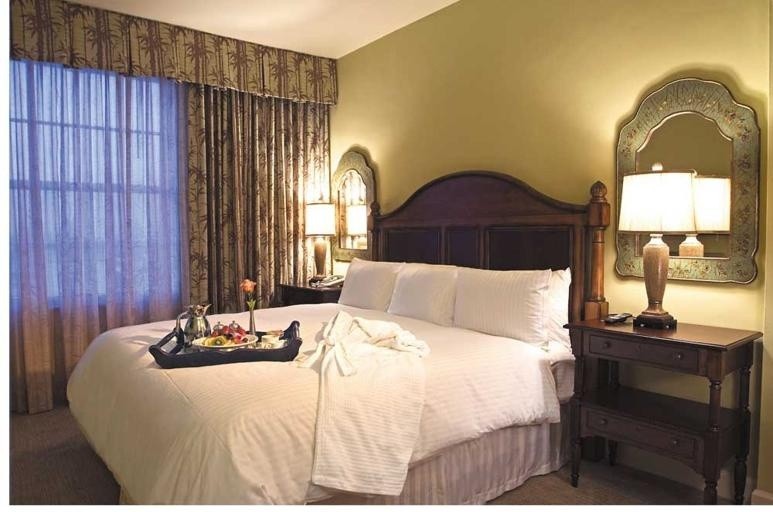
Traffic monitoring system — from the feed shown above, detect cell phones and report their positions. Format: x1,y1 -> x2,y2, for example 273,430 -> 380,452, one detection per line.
604,312 -> 632,324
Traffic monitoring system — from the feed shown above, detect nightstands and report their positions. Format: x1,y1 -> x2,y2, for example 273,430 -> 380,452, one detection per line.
275,282 -> 341,305
561,313 -> 763,504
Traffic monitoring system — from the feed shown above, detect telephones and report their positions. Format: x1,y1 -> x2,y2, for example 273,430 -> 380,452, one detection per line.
319,273 -> 345,289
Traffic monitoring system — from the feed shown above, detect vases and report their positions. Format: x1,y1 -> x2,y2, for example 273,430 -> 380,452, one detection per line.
248,310 -> 256,335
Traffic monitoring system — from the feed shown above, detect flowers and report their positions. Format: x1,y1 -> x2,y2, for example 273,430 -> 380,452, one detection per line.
238,278 -> 256,309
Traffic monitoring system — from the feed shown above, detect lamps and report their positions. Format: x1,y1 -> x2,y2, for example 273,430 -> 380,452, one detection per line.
344,205 -> 366,250
304,202 -> 336,283
617,170 -> 697,329
678,174 -> 731,258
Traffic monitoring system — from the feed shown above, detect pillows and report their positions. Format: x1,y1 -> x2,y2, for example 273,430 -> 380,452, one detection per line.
550,266 -> 572,342
453,267 -> 552,344
337,258 -> 402,311
387,263 -> 455,326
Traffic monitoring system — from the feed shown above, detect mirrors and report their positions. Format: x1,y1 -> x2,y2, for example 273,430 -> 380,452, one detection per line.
330,150 -> 375,262
613,78 -> 761,284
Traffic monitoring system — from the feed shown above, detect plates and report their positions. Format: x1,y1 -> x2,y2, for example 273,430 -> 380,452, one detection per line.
191,334 -> 258,348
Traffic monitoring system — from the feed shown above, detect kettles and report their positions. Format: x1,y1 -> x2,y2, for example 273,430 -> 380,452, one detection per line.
176,303 -> 212,346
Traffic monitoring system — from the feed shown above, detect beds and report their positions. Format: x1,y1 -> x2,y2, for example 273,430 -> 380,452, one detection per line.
66,171 -> 612,505
